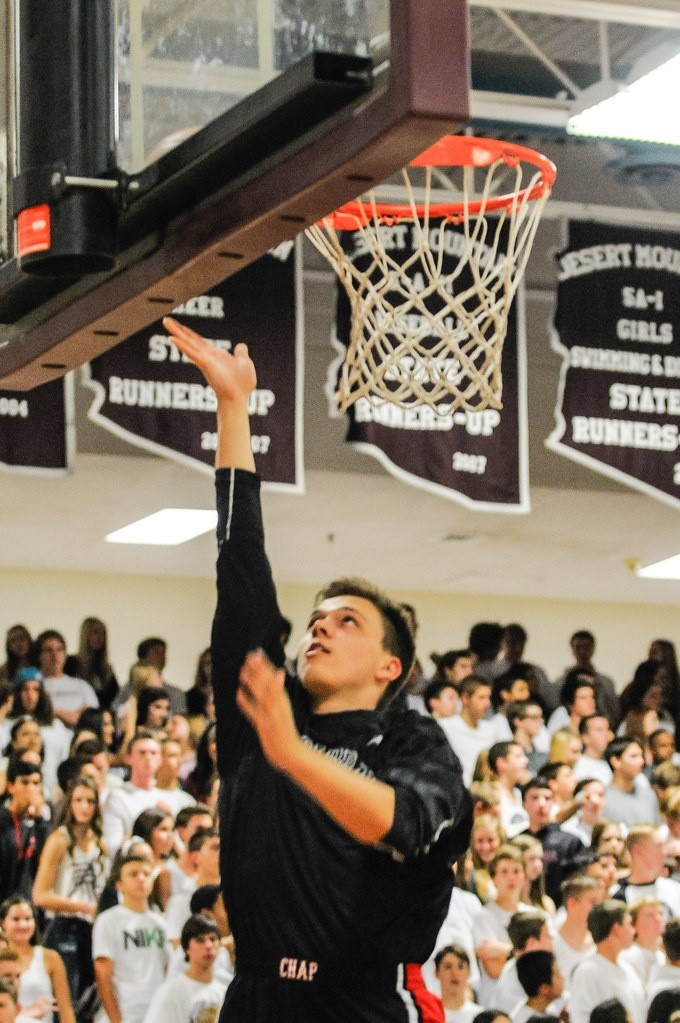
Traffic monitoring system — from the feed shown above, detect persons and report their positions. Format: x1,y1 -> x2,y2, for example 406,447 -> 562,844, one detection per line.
161,309 -> 469,1023
0,600 -> 680,1023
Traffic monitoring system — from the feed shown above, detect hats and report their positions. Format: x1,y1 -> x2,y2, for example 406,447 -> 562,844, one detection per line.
13,666 -> 42,686
